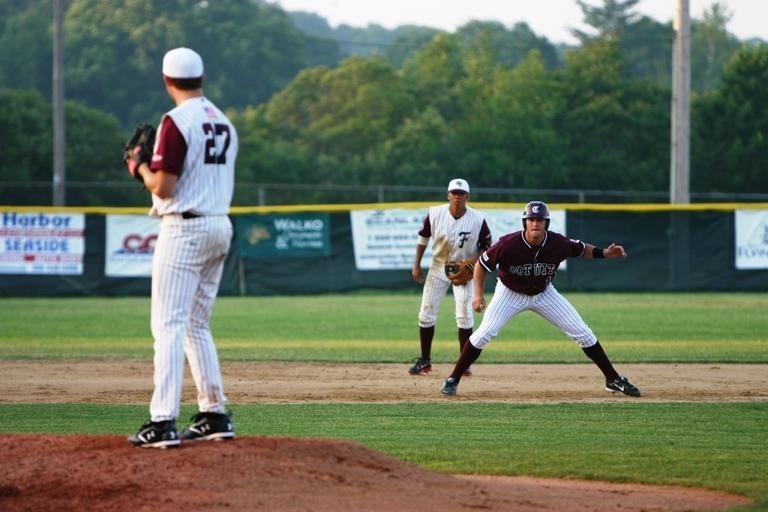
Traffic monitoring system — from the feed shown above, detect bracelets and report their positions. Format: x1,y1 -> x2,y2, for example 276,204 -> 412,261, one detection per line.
592,246 -> 606,258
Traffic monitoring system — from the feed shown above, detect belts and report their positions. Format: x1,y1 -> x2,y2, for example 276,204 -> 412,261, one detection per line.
181,210 -> 203,219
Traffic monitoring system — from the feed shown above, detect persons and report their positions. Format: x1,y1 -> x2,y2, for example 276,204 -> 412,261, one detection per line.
440,201 -> 641,399
124,46 -> 239,448
408,178 -> 492,377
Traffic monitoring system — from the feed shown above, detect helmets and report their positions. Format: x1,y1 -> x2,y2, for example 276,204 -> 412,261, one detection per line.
522,201 -> 550,230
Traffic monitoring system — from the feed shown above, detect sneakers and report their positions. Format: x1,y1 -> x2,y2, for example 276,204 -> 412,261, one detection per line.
179,411 -> 236,441
409,358 -> 432,374
440,377 -> 459,395
128,420 -> 181,448
605,376 -> 641,397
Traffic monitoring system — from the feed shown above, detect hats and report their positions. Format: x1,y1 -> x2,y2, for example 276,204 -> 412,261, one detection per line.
448,178 -> 470,194
162,46 -> 203,79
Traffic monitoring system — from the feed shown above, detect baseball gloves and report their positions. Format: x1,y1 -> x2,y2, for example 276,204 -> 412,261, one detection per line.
123,123 -> 155,184
445,255 -> 478,286
